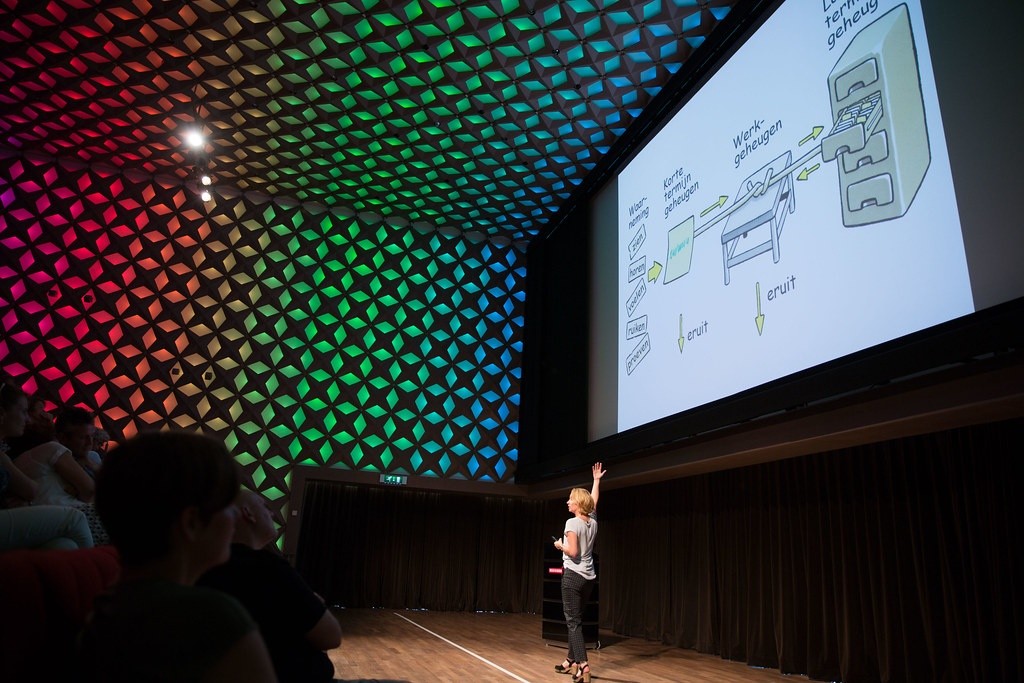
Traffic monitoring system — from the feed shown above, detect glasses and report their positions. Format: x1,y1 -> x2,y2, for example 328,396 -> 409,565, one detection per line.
0,377 -> 20,392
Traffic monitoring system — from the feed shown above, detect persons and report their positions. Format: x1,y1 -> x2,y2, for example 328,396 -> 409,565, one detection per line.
554,462 -> 607,683
0,382 -> 410,683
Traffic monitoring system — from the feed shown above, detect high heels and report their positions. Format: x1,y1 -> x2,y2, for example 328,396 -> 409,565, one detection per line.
555,659 -> 576,674
572,665 -> 591,683
